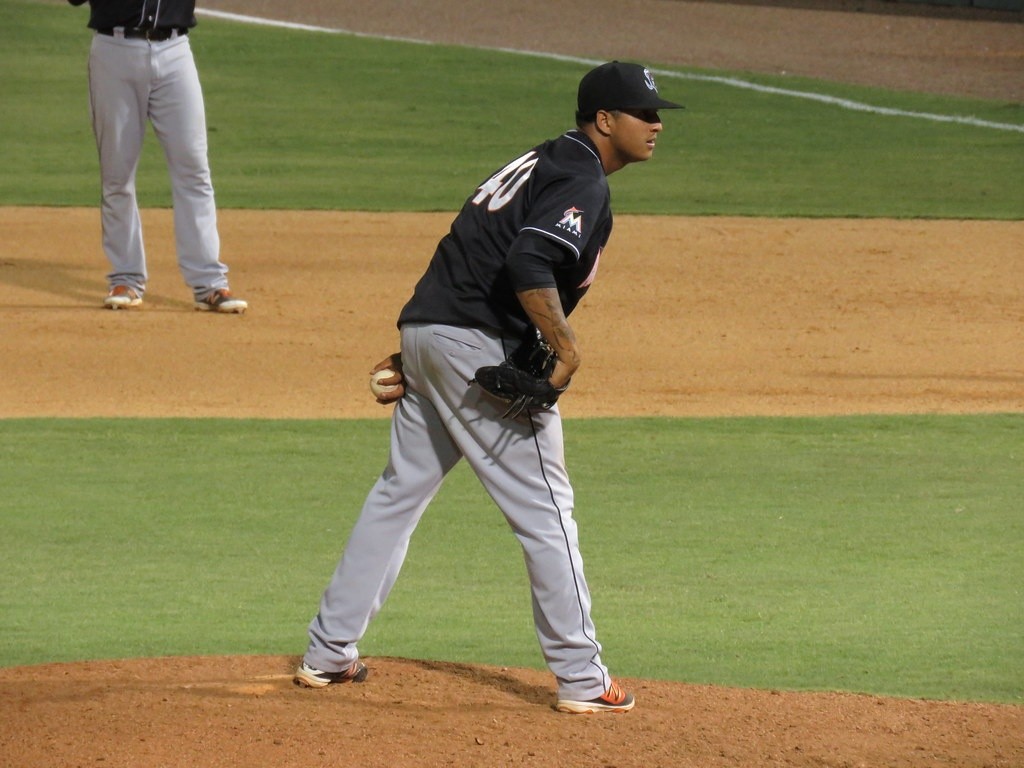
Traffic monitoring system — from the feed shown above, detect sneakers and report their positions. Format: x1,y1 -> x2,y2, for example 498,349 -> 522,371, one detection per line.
293,661 -> 367,688
104,286 -> 142,307
194,289 -> 248,312
557,681 -> 635,714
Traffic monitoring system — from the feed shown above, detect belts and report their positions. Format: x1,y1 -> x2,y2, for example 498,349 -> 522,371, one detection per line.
97,26 -> 188,42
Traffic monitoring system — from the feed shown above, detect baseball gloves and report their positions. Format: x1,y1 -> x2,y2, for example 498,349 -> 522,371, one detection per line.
467,338 -> 571,419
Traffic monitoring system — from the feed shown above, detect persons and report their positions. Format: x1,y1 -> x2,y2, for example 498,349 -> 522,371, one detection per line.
292,59 -> 685,714
67,0 -> 249,313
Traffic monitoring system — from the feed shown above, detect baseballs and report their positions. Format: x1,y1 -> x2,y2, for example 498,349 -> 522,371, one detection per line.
369,368 -> 401,399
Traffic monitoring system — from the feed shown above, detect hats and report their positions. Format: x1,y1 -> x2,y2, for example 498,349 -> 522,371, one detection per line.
578,60 -> 686,112
